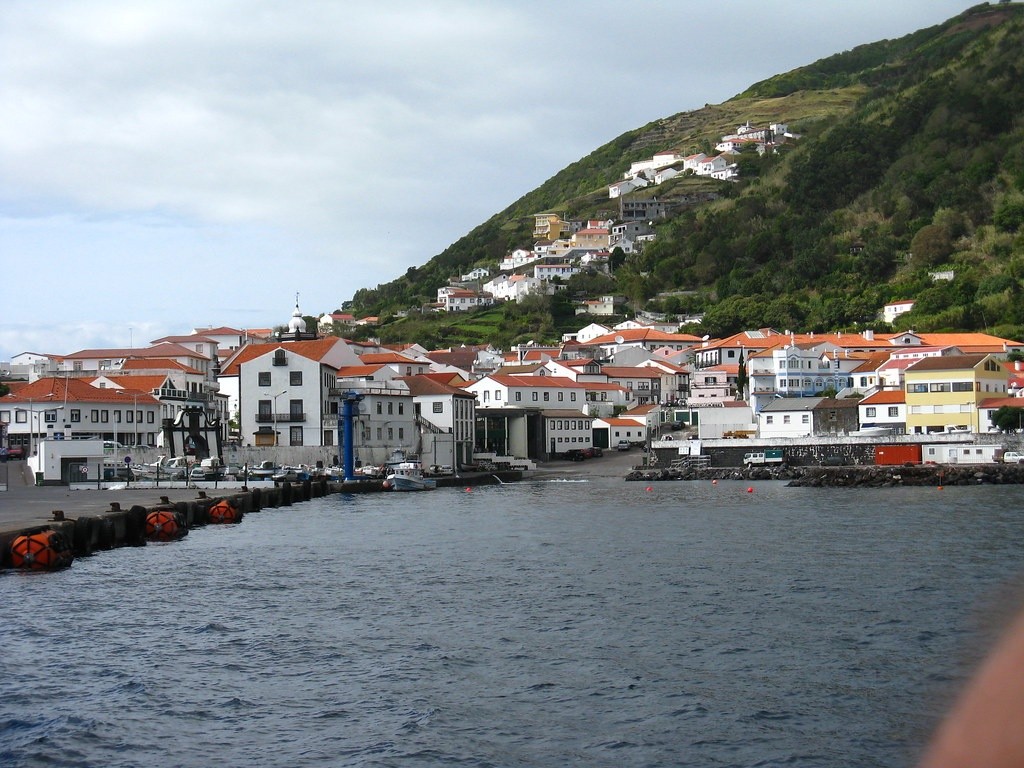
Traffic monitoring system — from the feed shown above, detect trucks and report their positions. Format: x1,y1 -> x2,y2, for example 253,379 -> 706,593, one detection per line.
991,448 -> 1024,464
743,452 -> 787,466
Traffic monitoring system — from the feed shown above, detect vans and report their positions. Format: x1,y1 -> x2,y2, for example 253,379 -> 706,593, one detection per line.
427,464 -> 454,476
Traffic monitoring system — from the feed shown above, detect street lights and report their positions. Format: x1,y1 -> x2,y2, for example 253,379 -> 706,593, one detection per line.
12,392 -> 54,458
14,406 -> 64,456
263,390 -> 287,468
117,389 -> 147,450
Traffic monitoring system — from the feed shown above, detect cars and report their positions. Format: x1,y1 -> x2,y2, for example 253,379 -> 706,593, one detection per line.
8,444 -> 27,460
271,470 -> 297,482
672,420 -> 686,430
0,448 -> 9,461
104,441 -> 152,451
561,446 -> 603,462
618,439 -> 632,451
190,469 -> 206,481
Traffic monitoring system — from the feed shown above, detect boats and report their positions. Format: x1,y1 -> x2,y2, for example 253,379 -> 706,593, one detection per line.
383,467 -> 437,492
930,424 -> 971,433
848,426 -> 894,436
131,456 -> 185,480
